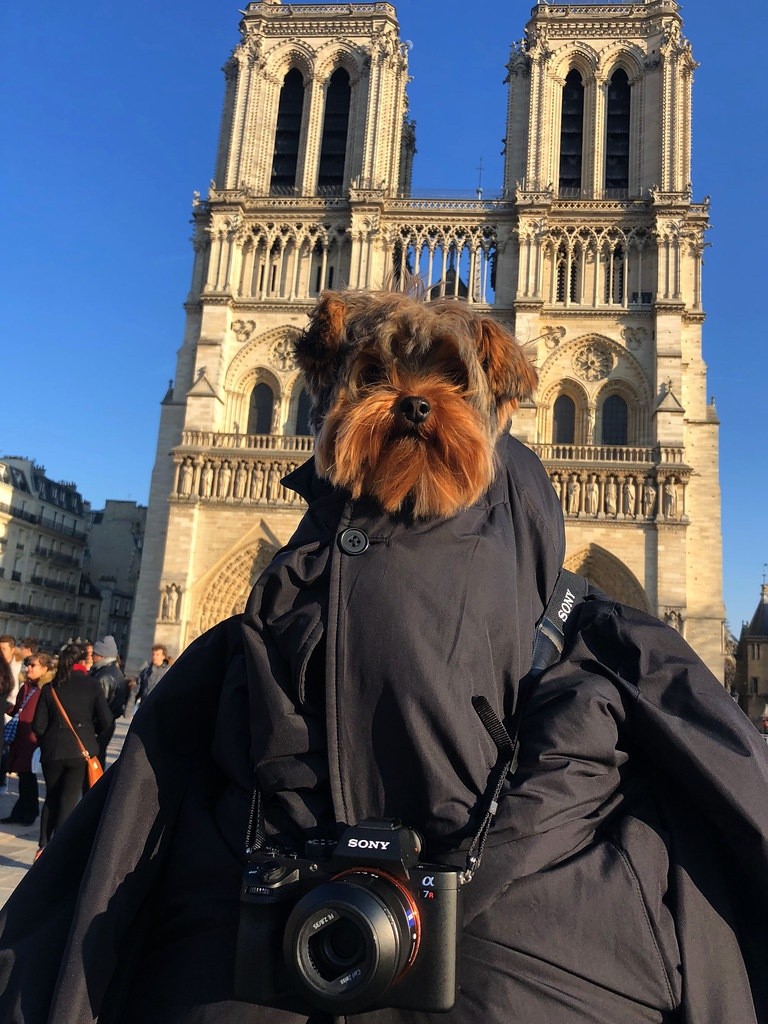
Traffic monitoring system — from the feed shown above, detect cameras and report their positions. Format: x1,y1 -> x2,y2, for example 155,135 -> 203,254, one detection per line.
236,816 -> 460,1013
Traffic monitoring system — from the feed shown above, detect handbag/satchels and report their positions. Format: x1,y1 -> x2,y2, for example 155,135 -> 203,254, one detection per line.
4,712 -> 19,745
86,755 -> 103,790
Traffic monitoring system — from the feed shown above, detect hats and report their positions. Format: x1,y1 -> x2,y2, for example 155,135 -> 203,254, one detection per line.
93,635 -> 117,658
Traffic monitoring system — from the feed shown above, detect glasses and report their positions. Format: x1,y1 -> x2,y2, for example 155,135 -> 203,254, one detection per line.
91,652 -> 102,658
26,663 -> 43,667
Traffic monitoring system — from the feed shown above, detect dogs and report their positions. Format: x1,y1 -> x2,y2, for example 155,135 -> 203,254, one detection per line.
287,259 -> 567,525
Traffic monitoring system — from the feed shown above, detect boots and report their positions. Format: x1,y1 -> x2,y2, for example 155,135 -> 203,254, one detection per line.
0,772 -> 40,827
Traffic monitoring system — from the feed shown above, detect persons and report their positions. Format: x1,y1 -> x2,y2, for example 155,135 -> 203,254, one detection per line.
30,644 -> 117,863
133,645 -> 173,709
0,635 -> 40,785
87,642 -> 126,765
1,653 -> 54,826
